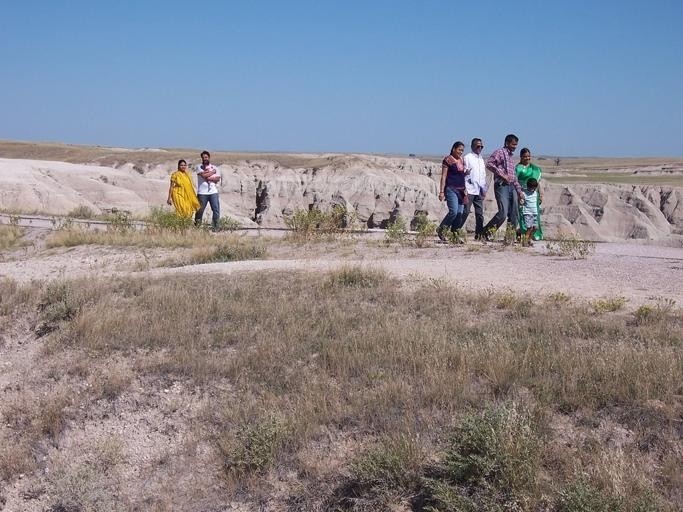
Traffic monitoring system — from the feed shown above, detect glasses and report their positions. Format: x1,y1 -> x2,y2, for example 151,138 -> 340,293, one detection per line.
477,145 -> 482,149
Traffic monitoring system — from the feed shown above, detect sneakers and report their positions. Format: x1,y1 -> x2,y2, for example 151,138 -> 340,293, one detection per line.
436,226 -> 448,241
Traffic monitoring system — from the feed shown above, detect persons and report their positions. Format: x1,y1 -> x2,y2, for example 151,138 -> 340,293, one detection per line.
435,142 -> 472,245
194,150 -> 221,232
458,138 -> 484,240
519,178 -> 542,247
201,159 -> 221,192
167,159 -> 203,218
515,148 -> 544,241
477,134 -> 525,246
